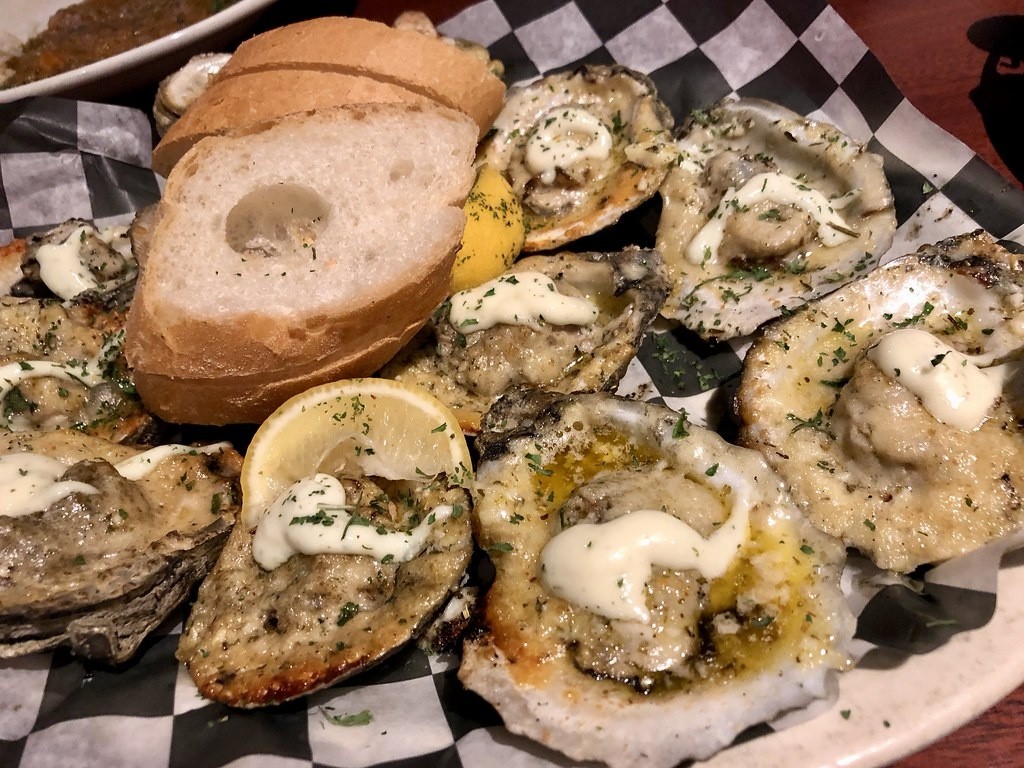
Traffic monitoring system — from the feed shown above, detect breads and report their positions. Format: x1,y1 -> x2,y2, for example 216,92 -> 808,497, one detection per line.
124,18 -> 507,426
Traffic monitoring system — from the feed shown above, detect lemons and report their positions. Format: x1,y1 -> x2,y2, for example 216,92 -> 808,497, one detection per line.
240,376 -> 475,527
449,161 -> 527,295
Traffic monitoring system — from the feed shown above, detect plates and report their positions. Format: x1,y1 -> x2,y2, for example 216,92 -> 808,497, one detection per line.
681,543 -> 1024,768
0,0 -> 277,105
